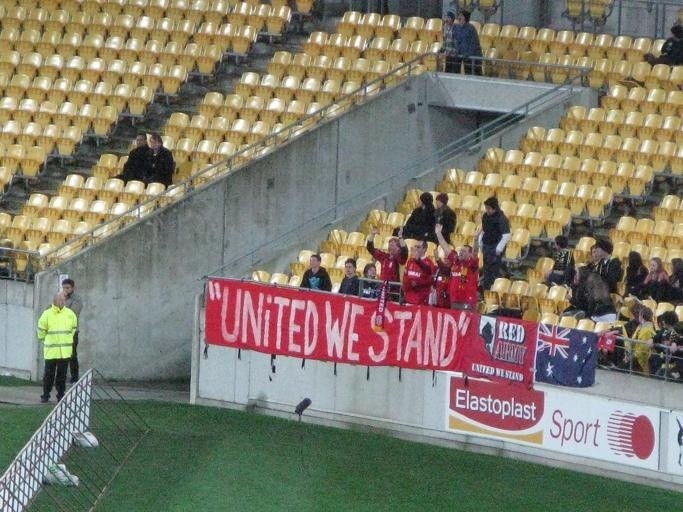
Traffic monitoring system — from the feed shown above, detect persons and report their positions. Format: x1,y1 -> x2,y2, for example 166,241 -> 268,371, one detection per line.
401,191 -> 457,246
454,8 -> 484,75
135,134 -> 175,186
56,278 -> 83,384
111,131 -> 148,180
642,23 -> 683,66
38,291 -> 74,403
435,10 -> 461,74
477,197 -> 511,290
302,224 -> 484,312
549,234 -> 681,384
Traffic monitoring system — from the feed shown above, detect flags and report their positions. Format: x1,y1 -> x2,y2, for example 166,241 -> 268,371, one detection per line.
535,320 -> 598,390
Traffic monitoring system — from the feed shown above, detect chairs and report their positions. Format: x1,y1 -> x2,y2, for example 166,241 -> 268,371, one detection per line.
0,0 -> 441,278
418,1 -> 682,379
